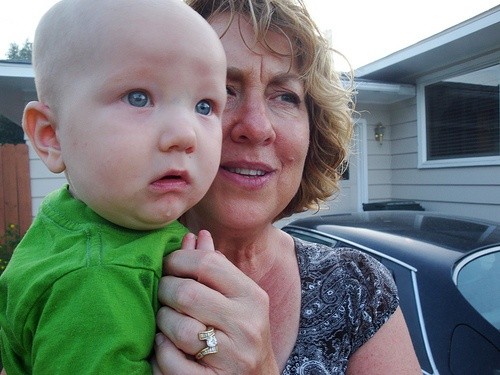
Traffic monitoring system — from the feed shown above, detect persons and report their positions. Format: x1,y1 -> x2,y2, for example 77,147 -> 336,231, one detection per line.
0,1 -> 227,375
152,0 -> 421,375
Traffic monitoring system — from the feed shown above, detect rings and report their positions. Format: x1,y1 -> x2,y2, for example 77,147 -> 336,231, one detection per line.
197,327 -> 218,360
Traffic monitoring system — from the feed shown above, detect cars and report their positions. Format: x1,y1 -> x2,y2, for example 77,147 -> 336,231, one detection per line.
280,211 -> 500,374
